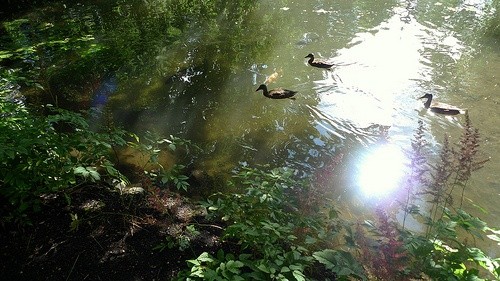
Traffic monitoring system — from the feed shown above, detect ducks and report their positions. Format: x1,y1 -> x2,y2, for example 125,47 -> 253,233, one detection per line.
255,84 -> 298,99
305,53 -> 335,70
417,93 -> 459,115
